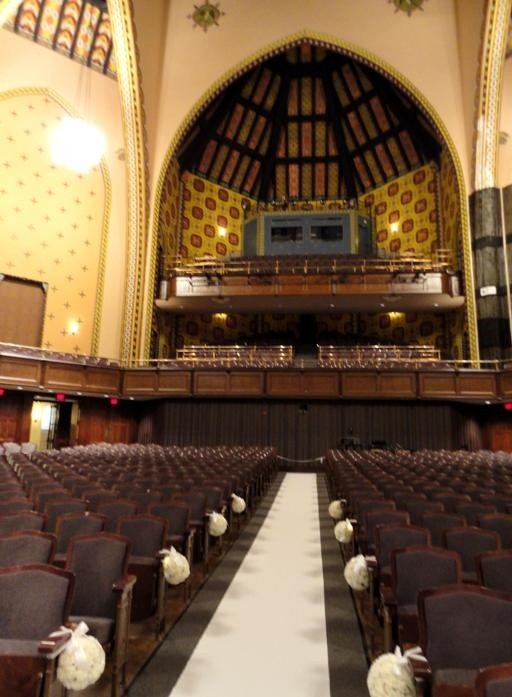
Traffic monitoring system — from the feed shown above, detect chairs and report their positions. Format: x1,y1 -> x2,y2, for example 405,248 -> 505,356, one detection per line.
1,343 -> 118,368
328,447 -> 512,697
159,344 -> 294,367
0,442 -> 279,697
162,249 -> 454,281
319,344 -> 450,368
